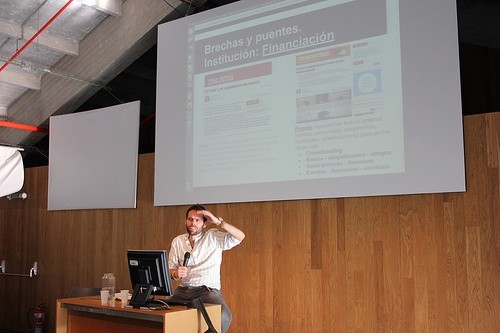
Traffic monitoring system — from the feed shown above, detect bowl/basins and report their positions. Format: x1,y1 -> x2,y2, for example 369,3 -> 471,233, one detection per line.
115,293 -> 132,300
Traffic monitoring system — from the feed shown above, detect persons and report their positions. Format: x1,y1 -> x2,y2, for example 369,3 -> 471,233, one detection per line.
168,204 -> 245,333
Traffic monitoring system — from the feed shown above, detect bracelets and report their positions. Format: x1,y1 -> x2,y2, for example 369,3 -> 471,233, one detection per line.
220,221 -> 224,228
172,271 -> 180,281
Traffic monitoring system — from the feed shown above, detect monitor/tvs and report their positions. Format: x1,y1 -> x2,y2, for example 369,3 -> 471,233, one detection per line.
127,249 -> 173,307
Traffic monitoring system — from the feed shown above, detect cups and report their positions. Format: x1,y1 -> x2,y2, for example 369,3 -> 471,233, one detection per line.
120,290 -> 129,303
100,291 -> 108,305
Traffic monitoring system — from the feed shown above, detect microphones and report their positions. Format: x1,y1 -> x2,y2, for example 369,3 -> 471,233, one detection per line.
183,252 -> 190,267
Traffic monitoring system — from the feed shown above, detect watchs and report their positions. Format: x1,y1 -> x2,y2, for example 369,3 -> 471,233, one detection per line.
216,217 -> 223,226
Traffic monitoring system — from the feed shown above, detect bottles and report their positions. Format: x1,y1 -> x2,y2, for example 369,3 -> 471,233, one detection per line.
102,273 -> 115,306
